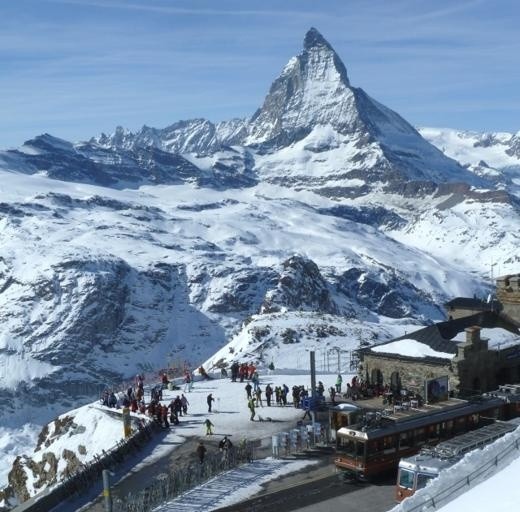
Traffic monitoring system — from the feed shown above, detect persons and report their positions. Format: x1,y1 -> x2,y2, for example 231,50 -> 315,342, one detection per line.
102,366 -> 215,428
202,418 -> 214,435
197,441 -> 208,466
245,378 -> 324,423
219,436 -> 233,464
220,361 -> 256,384
330,374 -> 422,410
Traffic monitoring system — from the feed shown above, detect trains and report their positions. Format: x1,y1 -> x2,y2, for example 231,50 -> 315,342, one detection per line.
331,381 -> 520,484
392,417 -> 520,504
